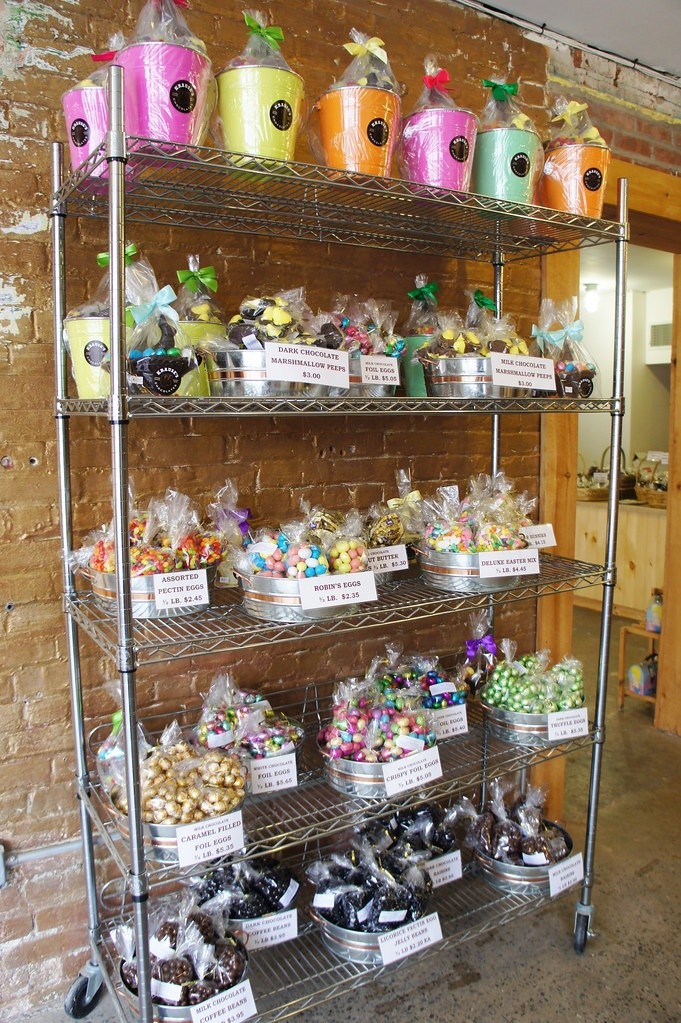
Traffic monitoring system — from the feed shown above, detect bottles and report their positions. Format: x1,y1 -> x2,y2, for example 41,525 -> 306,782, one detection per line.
647,587 -> 664,632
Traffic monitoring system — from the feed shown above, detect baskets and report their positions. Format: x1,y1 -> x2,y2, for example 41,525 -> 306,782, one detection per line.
578,445 -> 669,509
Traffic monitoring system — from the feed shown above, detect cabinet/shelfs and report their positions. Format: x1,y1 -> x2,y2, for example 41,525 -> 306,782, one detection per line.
575,501 -> 668,621
51,64 -> 631,1023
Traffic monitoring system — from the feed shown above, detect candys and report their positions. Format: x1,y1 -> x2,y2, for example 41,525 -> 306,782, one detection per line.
87,316 -> 595,1006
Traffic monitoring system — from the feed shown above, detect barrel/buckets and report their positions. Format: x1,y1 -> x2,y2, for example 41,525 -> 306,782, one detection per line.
59,43 -> 613,220
66,312 -> 502,401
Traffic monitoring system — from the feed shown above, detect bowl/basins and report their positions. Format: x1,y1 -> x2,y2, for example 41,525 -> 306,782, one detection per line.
86,562 -> 219,620
191,716 -> 306,786
475,681 -> 587,747
314,723 -> 438,799
305,871 -> 435,965
409,531 -> 530,594
419,828 -> 463,866
471,818 -> 574,898
116,928 -> 250,1021
186,888 -> 300,937
106,771 -> 249,863
230,566 -> 369,623
